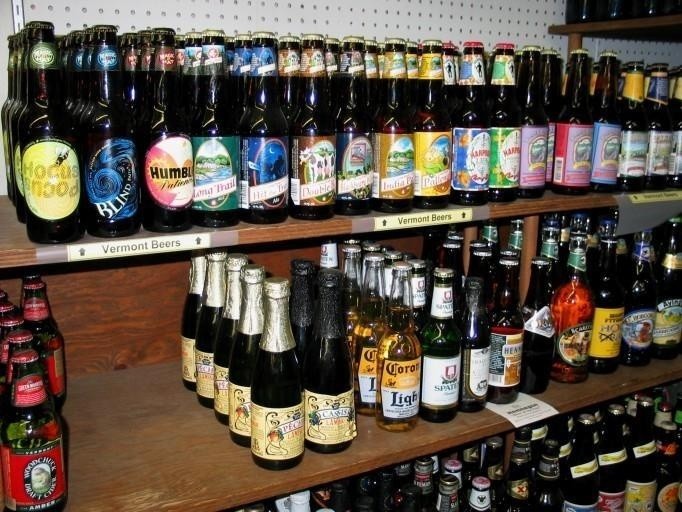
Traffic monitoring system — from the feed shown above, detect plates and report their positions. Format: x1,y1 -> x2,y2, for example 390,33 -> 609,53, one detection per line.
1,185 -> 682,511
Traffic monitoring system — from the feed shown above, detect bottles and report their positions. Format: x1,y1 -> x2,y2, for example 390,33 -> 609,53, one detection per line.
241,386 -> 681,512
182,211 -> 682,473
0,267 -> 67,511
0,18 -> 681,243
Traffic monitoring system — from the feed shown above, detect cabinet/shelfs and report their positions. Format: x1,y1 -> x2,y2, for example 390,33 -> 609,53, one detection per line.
0,267 -> 67,511
